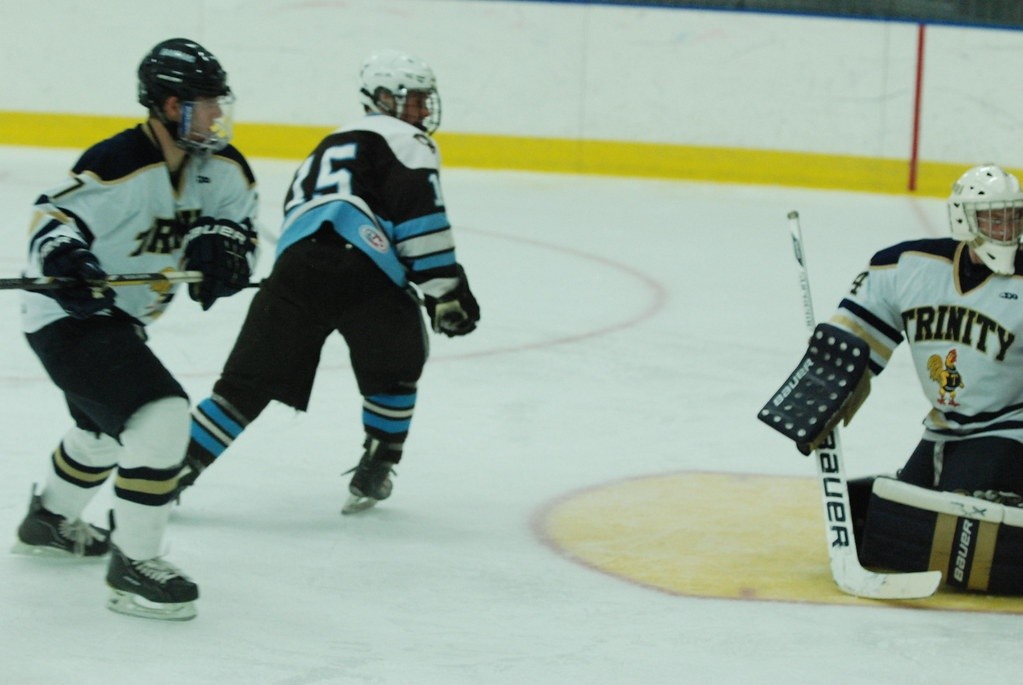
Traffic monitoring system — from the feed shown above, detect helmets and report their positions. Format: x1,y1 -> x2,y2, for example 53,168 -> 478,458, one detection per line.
138,37 -> 233,155
949,165 -> 1023,275
358,50 -> 440,136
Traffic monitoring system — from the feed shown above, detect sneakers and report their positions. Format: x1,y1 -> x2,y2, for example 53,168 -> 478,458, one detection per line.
10,483 -> 112,562
339,455 -> 398,514
106,542 -> 198,620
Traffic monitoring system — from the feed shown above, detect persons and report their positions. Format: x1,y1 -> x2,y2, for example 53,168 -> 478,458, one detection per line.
171,52 -> 480,515
17,38 -> 260,621
757,165 -> 1023,599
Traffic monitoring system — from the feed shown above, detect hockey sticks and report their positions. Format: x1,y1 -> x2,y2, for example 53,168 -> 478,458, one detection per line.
0,268 -> 206,290
786,208 -> 944,598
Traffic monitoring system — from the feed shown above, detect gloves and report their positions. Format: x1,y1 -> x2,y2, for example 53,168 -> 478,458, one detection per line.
39,235 -> 117,320
426,285 -> 481,338
183,217 -> 256,311
